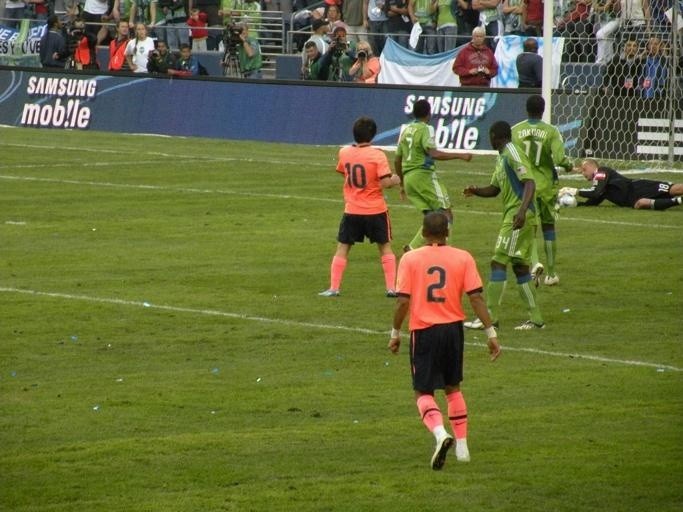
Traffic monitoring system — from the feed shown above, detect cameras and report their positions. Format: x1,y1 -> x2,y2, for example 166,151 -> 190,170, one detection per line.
356,48 -> 368,60
151,39 -> 160,58
478,64 -> 485,73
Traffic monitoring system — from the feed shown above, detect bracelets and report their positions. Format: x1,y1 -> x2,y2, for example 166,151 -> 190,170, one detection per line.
483,325 -> 498,339
390,328 -> 402,340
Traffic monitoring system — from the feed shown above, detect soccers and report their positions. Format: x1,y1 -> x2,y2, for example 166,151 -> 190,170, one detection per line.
558,187 -> 574,206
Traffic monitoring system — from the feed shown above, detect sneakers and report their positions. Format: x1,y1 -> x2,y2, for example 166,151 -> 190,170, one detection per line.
456,445 -> 471,463
530,262 -> 545,288
318,289 -> 341,297
387,289 -> 397,297
462,317 -> 500,331
513,320 -> 547,331
543,270 -> 560,287
402,243 -> 415,256
430,432 -> 455,471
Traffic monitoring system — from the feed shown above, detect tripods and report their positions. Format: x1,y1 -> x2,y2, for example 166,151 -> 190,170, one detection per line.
221,55 -> 247,78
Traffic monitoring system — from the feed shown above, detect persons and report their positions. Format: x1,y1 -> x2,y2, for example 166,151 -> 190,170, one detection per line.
319,115 -> 402,298
510,95 -> 574,288
3,1 -> 670,102
558,158 -> 683,211
394,95 -> 471,255
387,212 -> 502,470
462,120 -> 546,332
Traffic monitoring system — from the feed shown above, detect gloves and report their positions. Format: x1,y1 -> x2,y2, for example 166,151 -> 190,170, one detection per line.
557,185 -> 578,199
